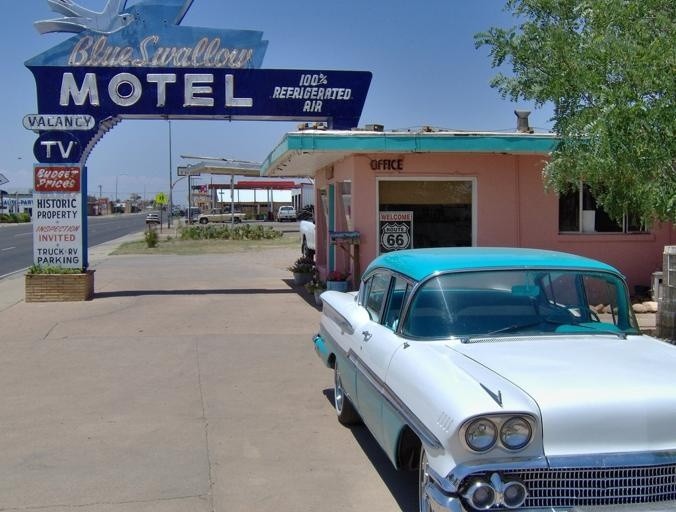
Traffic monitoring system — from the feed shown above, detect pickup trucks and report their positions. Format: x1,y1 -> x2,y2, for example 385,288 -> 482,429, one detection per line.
197,208 -> 247,224
312,247 -> 676,511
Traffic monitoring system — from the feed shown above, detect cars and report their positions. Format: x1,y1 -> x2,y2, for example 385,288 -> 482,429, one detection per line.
168,209 -> 185,217
145,213 -> 161,224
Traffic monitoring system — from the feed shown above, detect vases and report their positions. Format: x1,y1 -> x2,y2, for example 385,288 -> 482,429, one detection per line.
327,280 -> 350,293
314,289 -> 326,306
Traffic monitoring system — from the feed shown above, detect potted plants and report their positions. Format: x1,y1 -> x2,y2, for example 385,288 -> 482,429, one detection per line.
286,266 -> 314,285
294,256 -> 317,267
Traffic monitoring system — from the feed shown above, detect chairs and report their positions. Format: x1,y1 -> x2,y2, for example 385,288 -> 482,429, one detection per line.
381,293 -> 541,335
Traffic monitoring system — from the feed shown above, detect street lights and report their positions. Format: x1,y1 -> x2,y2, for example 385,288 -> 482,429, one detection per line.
115,173 -> 129,203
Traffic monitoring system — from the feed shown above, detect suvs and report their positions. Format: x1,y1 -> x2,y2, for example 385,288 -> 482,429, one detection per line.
277,206 -> 297,221
300,220 -> 316,264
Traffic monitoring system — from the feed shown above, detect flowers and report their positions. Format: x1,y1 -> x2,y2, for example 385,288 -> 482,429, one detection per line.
328,271 -> 351,281
304,280 -> 324,293
